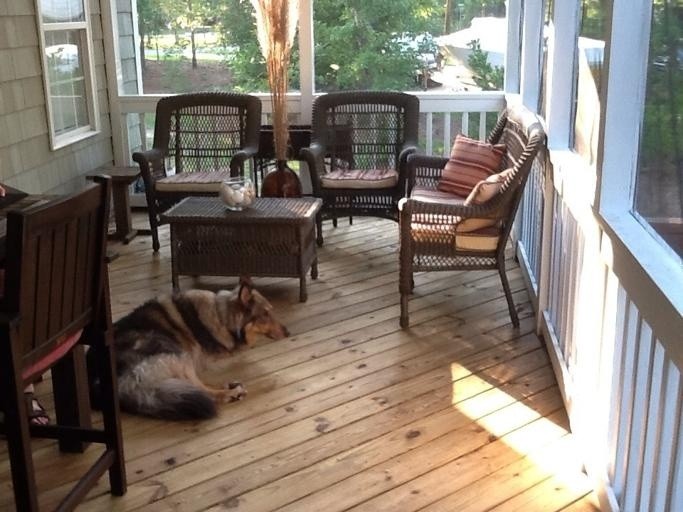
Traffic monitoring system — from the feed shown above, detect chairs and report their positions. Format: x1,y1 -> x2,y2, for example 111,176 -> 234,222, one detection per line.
140,92 -> 262,251
0,173 -> 128,511
300,91 -> 419,247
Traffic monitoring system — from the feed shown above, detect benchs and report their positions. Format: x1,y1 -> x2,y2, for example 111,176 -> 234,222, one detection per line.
397,102 -> 543,329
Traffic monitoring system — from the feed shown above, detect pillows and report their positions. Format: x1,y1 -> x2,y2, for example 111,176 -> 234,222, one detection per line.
437,136 -> 516,232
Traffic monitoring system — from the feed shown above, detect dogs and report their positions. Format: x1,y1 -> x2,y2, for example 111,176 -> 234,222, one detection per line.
84,270 -> 291,423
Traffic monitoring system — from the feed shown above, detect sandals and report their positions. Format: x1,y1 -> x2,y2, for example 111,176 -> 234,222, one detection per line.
23,392 -> 51,425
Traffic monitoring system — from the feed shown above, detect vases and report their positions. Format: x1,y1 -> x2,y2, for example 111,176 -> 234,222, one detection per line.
262,158 -> 302,198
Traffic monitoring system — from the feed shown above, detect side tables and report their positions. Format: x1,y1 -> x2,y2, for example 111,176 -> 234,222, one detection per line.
84,164 -> 142,245
